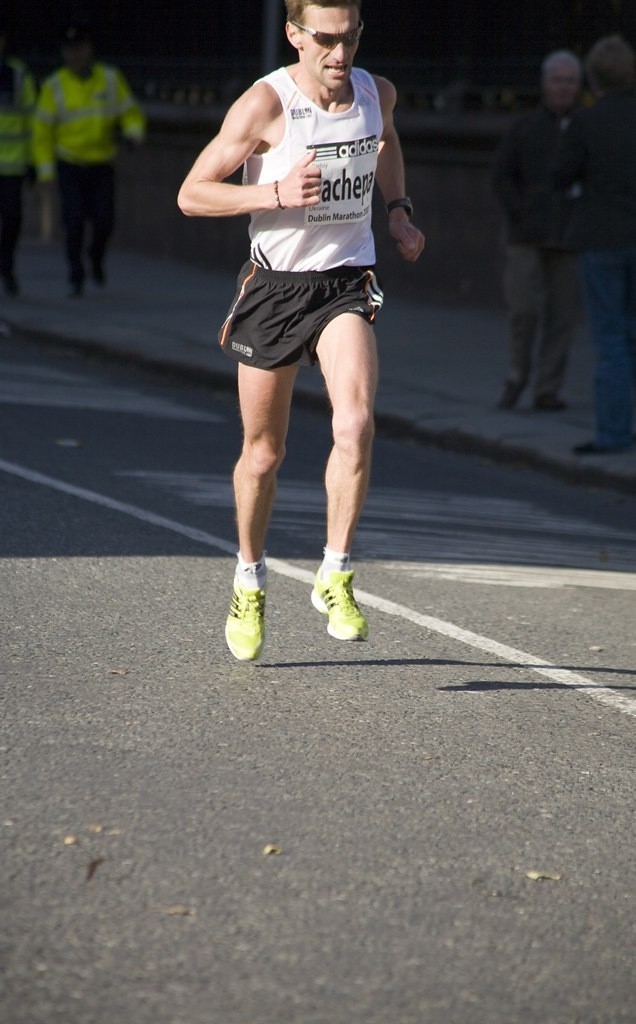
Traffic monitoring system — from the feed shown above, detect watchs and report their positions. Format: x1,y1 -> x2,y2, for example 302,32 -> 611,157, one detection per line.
387,198 -> 413,217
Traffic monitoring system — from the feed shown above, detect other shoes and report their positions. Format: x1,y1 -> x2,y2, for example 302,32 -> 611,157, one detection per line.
3,274 -> 17,294
71,276 -> 85,297
497,380 -> 528,411
570,443 -> 606,455
535,393 -> 566,410
90,261 -> 105,285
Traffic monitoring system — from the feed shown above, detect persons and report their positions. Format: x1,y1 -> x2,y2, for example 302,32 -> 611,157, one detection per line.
0,32 -> 37,295
547,34 -> 636,453
491,49 -> 583,413
30,33 -> 146,297
177,0 -> 458,660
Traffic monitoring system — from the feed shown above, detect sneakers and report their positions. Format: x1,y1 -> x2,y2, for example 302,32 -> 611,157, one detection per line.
225,575 -> 266,662
309,570 -> 367,641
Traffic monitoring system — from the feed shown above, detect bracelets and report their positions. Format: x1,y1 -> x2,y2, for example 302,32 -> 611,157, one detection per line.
274,180 -> 285,210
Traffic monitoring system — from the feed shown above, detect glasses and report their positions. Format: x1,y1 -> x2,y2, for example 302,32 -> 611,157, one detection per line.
292,19 -> 366,49
